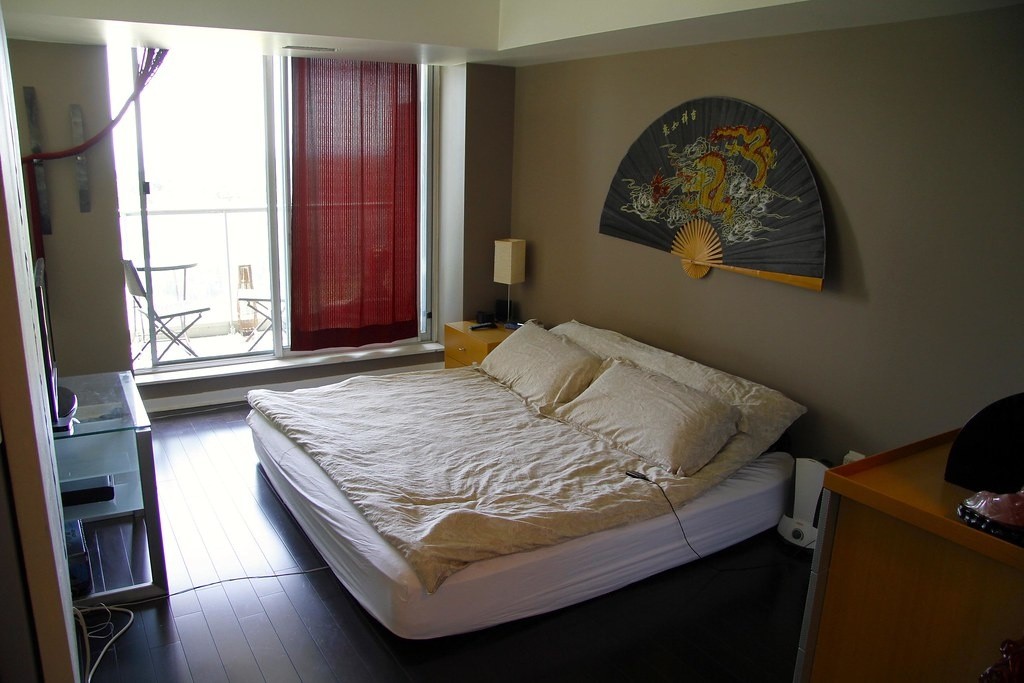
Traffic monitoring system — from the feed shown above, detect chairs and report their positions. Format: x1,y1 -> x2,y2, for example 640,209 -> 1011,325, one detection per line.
238,288 -> 288,352
121,259 -> 210,363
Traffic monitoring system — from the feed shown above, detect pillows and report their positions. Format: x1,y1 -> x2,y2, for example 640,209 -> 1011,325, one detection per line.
474,318 -> 613,418
538,355 -> 743,478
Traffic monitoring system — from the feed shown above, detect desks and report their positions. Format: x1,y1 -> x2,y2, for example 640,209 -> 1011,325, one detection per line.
136,262 -> 200,355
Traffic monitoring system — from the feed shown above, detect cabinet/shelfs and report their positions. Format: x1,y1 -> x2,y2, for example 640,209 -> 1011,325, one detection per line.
57,369 -> 171,611
789,428 -> 1024,683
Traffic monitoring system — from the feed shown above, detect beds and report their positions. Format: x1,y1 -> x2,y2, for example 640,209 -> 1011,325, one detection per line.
246,319 -> 808,666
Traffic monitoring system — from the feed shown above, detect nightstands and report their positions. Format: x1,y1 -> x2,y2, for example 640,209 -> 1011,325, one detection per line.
444,319 -> 516,369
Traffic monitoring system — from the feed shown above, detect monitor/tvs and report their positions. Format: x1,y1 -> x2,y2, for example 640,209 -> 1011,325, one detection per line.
32,258 -> 78,426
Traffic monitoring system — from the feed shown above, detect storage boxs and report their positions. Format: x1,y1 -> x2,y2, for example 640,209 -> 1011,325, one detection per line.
62,519 -> 85,558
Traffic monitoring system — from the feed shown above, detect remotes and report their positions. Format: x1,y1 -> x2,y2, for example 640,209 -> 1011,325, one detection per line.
469,323 -> 497,330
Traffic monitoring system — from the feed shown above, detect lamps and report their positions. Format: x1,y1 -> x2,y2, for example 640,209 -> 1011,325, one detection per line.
493,239 -> 528,327
